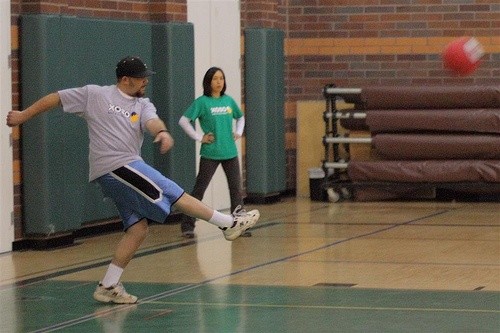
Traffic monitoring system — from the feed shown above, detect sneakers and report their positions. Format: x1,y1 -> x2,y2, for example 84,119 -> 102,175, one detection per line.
241,231 -> 252,237
94,281 -> 138,304
184,231 -> 194,238
221,209 -> 260,241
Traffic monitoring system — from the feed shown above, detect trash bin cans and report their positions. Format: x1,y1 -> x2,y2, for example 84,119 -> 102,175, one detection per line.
309,168 -> 334,202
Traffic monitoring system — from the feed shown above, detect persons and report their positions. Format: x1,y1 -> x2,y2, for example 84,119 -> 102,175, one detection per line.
6,56 -> 260,304
177,67 -> 252,238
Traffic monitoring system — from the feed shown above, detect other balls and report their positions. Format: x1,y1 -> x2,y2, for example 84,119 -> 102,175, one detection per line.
443,39 -> 485,77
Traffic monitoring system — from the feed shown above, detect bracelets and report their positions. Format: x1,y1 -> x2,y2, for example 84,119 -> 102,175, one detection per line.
157,130 -> 170,134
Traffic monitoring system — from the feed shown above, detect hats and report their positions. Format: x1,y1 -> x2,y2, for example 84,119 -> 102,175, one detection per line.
115,56 -> 156,77
